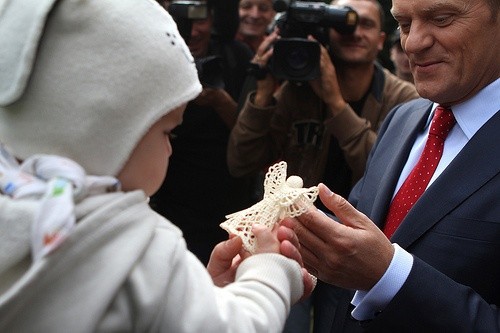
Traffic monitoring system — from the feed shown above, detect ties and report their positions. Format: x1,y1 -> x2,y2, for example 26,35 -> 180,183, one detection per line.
384,107 -> 456,238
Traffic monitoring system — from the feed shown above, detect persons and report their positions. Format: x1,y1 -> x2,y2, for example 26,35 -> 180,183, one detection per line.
287,0 -> 499,332
227,0 -> 421,219
233,0 -> 282,58
390,26 -> 417,86
0,0 -> 314,333
148,0 -> 259,267
